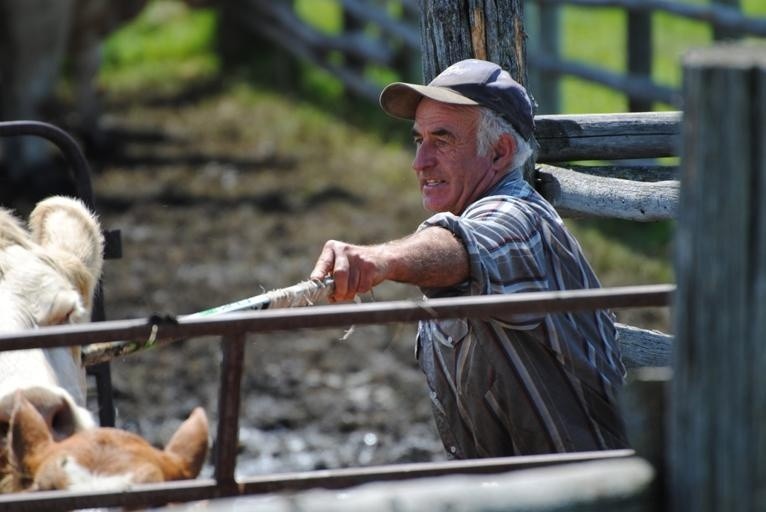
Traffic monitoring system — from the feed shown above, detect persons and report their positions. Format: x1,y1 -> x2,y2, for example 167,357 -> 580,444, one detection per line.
309,57 -> 632,460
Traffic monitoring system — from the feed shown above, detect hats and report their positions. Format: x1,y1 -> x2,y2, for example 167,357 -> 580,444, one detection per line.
379,60 -> 536,141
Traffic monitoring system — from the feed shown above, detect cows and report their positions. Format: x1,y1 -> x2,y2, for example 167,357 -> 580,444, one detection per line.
0,195 -> 106,479
0,389 -> 209,512
